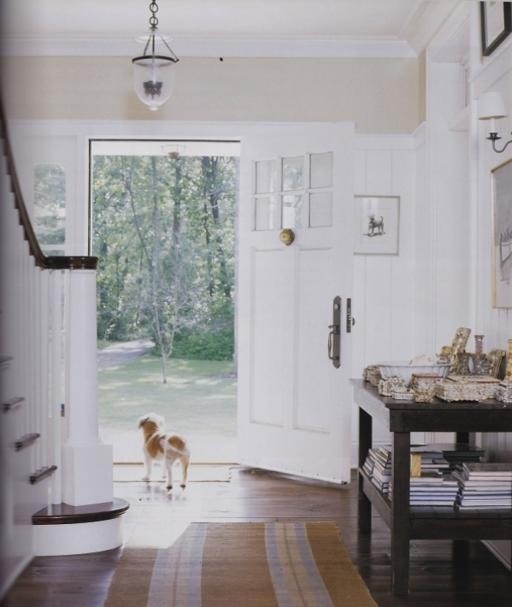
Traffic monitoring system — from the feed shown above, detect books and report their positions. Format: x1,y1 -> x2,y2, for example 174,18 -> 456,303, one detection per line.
360,440 -> 510,508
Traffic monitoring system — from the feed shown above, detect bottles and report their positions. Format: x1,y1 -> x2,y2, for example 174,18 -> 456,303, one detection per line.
438,326 -> 512,385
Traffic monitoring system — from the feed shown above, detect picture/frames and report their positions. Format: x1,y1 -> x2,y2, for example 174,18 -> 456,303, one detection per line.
354,195 -> 400,256
487,155 -> 512,308
481,0 -> 511,57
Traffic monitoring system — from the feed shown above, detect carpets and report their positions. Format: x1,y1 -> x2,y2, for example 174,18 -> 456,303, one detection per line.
111,462 -> 235,483
102,521 -> 377,606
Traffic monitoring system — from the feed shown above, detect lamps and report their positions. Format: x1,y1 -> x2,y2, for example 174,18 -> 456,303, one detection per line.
478,92 -> 511,154
132,0 -> 180,114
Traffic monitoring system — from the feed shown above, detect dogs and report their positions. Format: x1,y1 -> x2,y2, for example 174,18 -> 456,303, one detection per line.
137,412 -> 192,490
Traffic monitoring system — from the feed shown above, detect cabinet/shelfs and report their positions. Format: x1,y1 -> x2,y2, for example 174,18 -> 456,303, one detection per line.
351,378 -> 512,595
